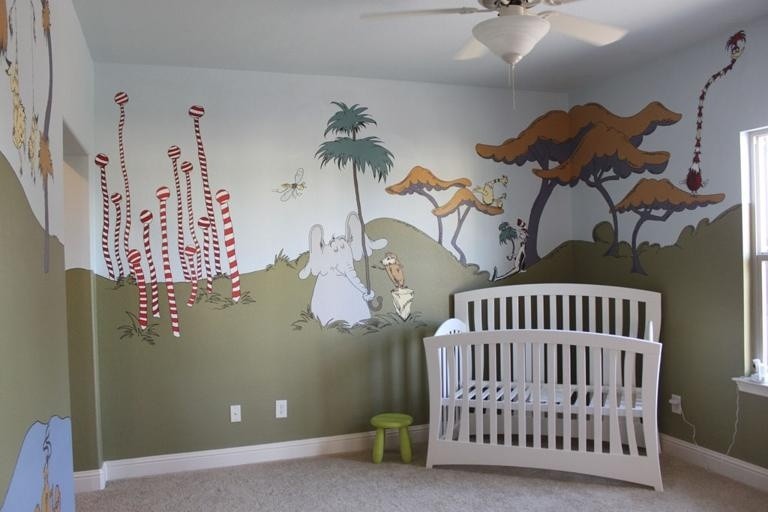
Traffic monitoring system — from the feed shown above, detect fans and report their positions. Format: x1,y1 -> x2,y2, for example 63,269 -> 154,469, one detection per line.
360,0 -> 627,62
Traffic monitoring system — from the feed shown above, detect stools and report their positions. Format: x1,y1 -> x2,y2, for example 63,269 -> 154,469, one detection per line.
371,413 -> 413,463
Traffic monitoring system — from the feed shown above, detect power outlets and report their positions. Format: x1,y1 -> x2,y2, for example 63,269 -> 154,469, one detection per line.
669,394 -> 681,414
275,400 -> 287,418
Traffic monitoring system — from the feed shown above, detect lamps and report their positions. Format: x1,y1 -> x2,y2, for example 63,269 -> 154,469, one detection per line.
472,15 -> 551,68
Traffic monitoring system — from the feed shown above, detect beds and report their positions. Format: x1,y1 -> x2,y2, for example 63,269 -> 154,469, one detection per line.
423,282 -> 662,491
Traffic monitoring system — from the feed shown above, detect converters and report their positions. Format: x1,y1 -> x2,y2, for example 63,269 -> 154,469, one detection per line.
668,398 -> 681,406
